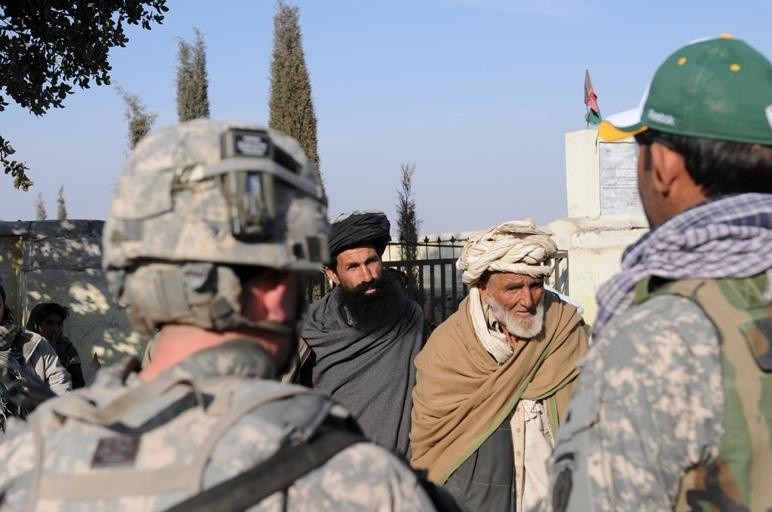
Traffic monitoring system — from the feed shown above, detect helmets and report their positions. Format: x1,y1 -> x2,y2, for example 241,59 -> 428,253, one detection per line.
99,119 -> 330,333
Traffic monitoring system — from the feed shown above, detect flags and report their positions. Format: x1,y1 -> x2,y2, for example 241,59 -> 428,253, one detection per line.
585,72 -> 601,124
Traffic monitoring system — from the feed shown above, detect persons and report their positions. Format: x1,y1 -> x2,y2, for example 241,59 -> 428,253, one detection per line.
412,214 -> 593,510
0,285 -> 74,427
25,300 -> 85,393
548,32 -> 772,510
0,119 -> 436,511
290,202 -> 435,468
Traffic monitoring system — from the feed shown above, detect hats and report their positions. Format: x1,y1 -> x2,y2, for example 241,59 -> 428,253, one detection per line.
322,209 -> 391,263
454,217 -> 558,288
598,36 -> 768,148
